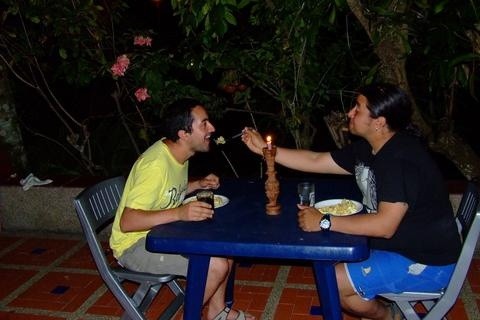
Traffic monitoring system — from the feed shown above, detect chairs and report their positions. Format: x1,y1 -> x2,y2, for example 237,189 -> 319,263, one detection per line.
74,175 -> 185,320
376,177 -> 480,319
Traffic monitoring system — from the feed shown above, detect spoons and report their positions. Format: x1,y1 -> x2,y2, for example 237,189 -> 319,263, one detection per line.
212,126 -> 253,145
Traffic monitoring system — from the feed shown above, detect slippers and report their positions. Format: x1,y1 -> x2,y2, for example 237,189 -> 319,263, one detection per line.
212,306 -> 256,320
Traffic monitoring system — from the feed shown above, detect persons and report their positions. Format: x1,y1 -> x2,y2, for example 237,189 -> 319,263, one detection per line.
108,99 -> 254,320
241,83 -> 462,320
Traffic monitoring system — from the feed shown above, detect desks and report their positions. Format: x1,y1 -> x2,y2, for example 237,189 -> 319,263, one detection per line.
146,180 -> 372,320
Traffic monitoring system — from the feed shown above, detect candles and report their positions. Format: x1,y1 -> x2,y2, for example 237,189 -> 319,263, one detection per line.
266,135 -> 273,150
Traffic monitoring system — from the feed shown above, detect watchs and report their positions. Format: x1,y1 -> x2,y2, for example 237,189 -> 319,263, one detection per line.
319,213 -> 332,233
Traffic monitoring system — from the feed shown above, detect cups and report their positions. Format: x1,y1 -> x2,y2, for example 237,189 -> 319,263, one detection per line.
298,180 -> 315,209
196,190 -> 216,222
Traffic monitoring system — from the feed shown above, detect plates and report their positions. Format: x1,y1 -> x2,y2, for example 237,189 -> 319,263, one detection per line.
314,199 -> 365,217
183,194 -> 230,209
